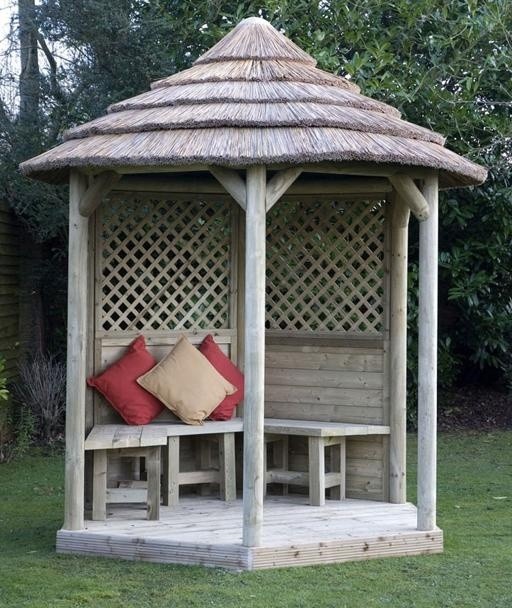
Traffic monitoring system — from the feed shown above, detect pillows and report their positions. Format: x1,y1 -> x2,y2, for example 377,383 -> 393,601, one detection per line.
87,334 -> 165,425
196,335 -> 244,421
136,337 -> 238,425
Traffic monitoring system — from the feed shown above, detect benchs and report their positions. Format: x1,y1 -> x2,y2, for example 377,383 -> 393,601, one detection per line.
84,416 -> 390,521
